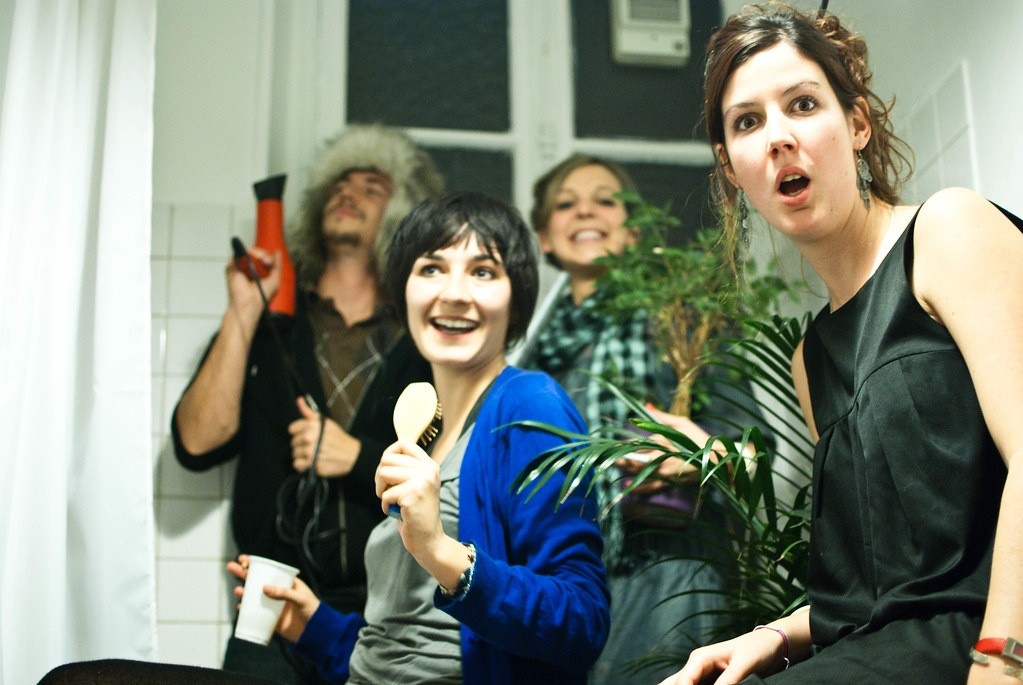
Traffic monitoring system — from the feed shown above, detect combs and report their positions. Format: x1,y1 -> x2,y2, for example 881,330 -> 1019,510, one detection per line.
386,381 -> 443,521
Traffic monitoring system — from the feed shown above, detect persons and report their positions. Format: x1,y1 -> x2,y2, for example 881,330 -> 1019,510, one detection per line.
515,152 -> 777,685
36,190 -> 611,685
648,0 -> 1023,685
167,123 -> 452,674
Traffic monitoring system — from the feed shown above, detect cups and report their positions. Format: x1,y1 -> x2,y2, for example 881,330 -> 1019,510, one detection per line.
235,555 -> 299,646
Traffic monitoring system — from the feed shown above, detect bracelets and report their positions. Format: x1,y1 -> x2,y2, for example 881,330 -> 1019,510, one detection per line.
752,623 -> 791,671
969,636 -> 1023,679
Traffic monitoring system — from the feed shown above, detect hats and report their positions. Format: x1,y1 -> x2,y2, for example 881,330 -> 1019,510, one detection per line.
285,122 -> 447,281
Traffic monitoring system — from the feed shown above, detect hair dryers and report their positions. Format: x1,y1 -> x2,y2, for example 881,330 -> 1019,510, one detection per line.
231,173 -> 296,333
